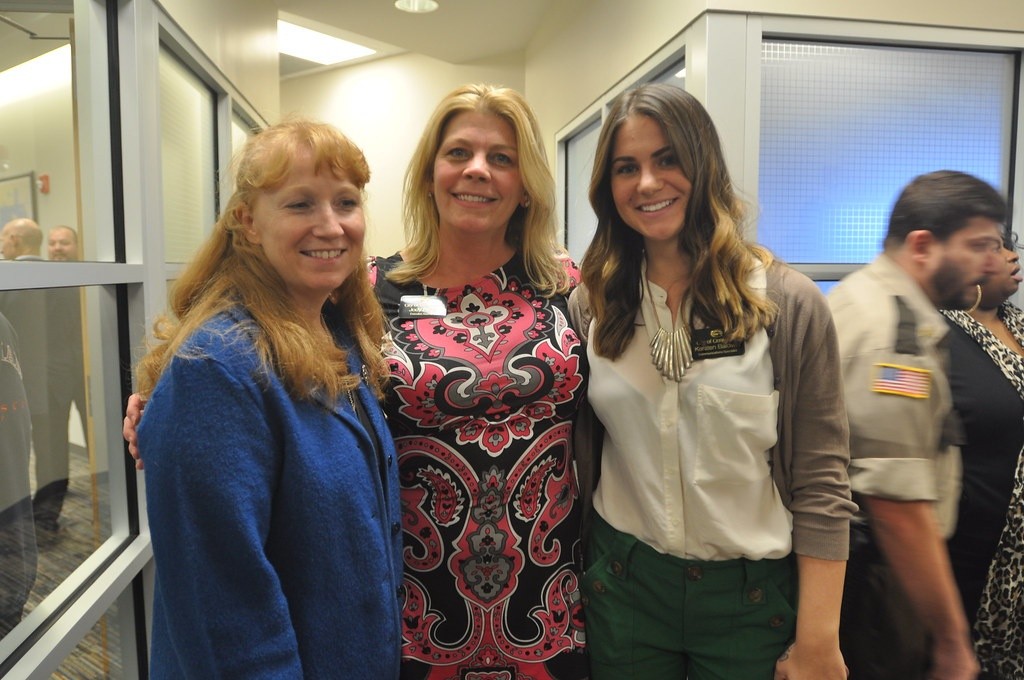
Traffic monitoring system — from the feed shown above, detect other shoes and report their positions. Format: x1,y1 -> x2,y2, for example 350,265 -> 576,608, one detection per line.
35,520 -> 59,548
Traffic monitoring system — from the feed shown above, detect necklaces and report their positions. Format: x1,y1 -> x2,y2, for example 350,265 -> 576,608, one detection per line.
321,316 -> 356,411
644,263 -> 696,382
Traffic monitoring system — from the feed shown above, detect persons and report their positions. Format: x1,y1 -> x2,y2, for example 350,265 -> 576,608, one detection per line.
568,82 -> 859,680
0,219 -> 86,637
823,169 -> 1024,680
123,81 -> 588,680
121,120 -> 405,680
